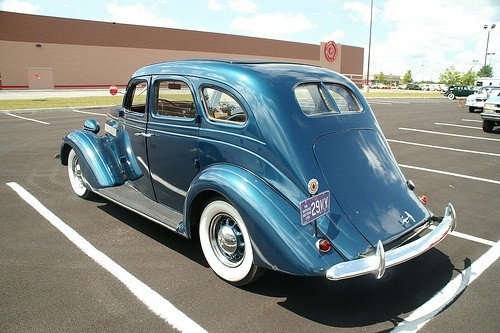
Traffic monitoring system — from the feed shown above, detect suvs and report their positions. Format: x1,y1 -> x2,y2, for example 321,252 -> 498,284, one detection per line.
465,87 -> 500,132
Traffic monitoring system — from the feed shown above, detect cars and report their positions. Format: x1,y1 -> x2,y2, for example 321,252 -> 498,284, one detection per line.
443,85 -> 478,100
371,82 -> 403,89
53,59 -> 456,287
406,83 -> 448,92
